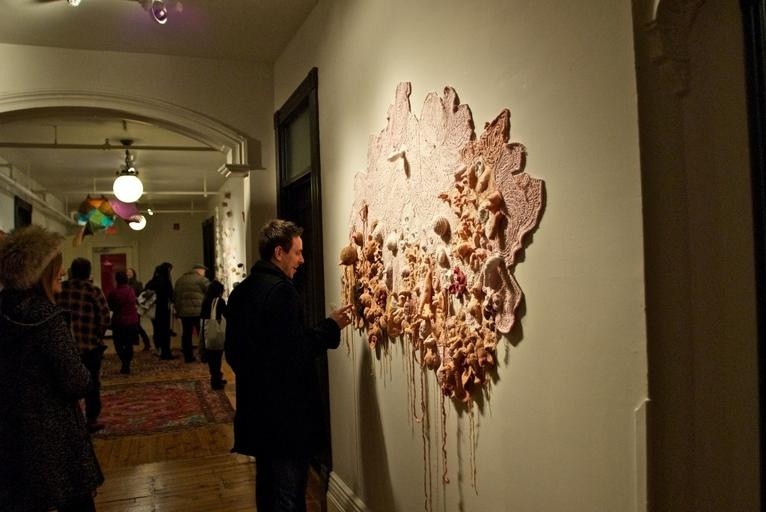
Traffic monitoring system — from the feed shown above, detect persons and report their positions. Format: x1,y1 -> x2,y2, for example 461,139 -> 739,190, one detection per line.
173,262 -> 213,363
53,256 -> 114,433
107,270 -> 139,375
143,263 -> 178,359
221,217 -> 352,511
124,269 -> 151,352
200,280 -> 227,389
0,224 -> 105,512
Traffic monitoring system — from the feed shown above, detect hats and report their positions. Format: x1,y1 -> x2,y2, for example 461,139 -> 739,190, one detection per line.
0,223 -> 67,292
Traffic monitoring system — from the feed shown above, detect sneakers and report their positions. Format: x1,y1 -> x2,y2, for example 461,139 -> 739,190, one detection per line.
119,344 -> 228,392
86,421 -> 106,434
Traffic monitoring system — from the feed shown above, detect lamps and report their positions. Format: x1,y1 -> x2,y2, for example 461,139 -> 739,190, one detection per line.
112,138 -> 144,203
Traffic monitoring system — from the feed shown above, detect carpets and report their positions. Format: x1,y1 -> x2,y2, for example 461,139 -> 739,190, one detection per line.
78,345 -> 236,440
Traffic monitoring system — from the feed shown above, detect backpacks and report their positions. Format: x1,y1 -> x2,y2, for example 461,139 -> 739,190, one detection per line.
133,288 -> 158,321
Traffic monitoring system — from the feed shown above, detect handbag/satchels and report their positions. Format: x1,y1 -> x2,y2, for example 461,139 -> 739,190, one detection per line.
199,298 -> 227,352
